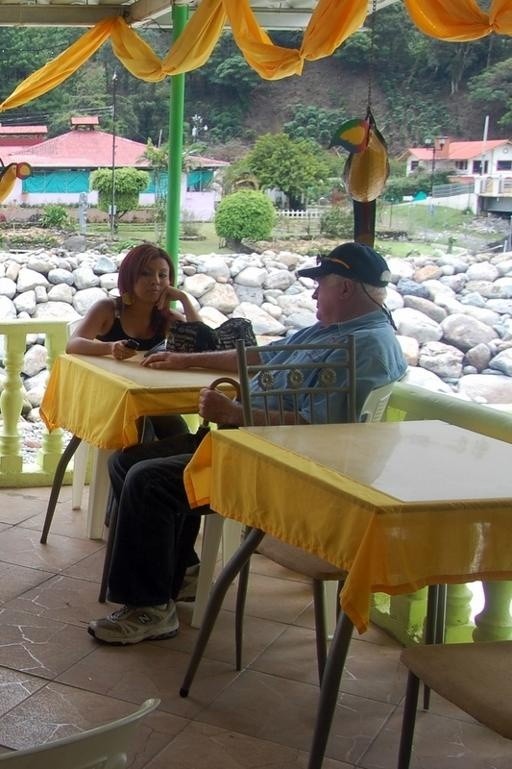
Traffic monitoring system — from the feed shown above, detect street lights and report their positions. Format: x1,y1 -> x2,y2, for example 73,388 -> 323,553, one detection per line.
190,115 -> 208,143
109,71 -> 120,235
424,134 -> 447,196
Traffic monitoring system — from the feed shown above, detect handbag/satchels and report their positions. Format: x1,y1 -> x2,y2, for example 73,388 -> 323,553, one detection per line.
167,316 -> 259,354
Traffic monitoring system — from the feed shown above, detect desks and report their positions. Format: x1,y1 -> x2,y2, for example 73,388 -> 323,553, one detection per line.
41,351 -> 254,603
179,419 -> 512,769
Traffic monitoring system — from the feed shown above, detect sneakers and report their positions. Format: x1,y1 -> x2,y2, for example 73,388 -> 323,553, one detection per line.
169,569 -> 216,602
87,599 -> 180,645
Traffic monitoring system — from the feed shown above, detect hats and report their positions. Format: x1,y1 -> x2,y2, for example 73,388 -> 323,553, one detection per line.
295,240 -> 390,287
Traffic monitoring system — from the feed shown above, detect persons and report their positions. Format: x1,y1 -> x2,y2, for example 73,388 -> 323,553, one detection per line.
65,245 -> 202,447
87,241 -> 410,646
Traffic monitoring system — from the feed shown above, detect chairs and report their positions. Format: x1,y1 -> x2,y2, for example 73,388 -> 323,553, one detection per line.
67,317 -> 117,541
234,332 -> 442,709
395,638 -> 512,768
0,696 -> 162,768
190,378 -> 400,631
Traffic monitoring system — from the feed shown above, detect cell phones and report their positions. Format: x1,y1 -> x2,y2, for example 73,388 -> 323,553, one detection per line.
125,339 -> 140,350
